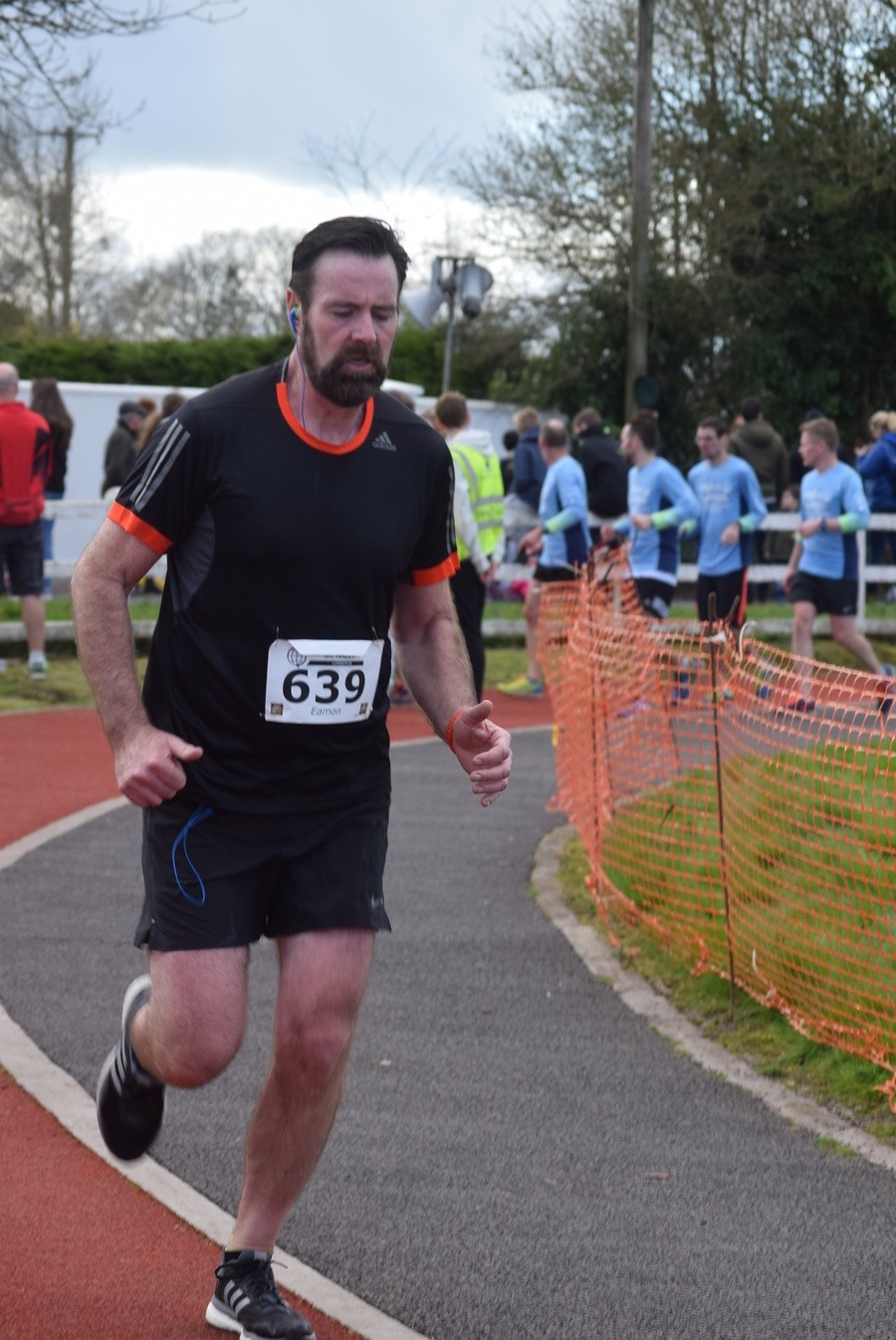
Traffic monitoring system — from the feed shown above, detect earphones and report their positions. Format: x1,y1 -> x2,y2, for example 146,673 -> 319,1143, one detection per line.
289,305 -> 300,334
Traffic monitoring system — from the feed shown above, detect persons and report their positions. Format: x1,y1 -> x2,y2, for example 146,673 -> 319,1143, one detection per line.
98,393 -> 187,596
73,216 -> 513,1340
0,360 -> 74,685
388,392 -> 896,720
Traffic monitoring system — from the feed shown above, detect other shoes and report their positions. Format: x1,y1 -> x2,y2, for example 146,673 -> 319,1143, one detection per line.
387,676 -> 413,704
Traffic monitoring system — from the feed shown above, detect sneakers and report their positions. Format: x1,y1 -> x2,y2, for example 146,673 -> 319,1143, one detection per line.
876,665 -> 896,715
206,1247 -> 316,1340
23,658 -> 48,681
701,683 -> 741,704
96,973 -> 169,1163
618,697 -> 653,722
670,658 -> 704,708
774,697 -> 818,715
494,674 -> 545,702
754,662 -> 775,702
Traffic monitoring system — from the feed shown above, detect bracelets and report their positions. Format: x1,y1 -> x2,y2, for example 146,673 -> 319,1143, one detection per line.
821,519 -> 826,531
447,708 -> 462,755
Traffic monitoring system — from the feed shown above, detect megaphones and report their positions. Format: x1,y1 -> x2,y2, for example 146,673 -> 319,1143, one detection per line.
400,276 -> 447,327
450,264 -> 494,318
